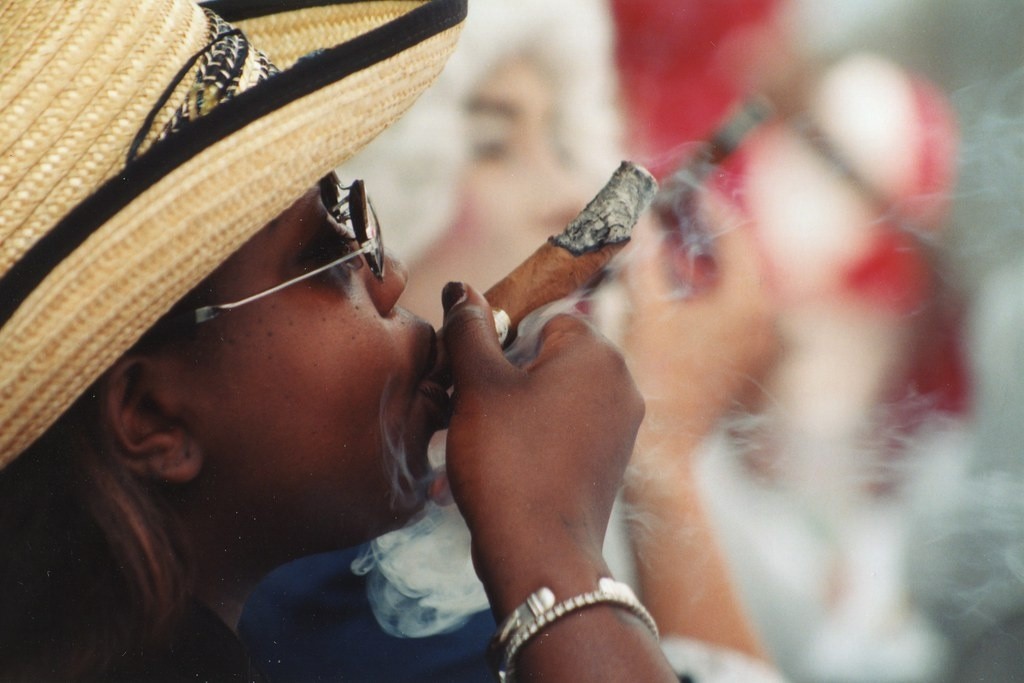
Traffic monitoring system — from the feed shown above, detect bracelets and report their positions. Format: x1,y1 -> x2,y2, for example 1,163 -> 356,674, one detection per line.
489,580 -> 658,680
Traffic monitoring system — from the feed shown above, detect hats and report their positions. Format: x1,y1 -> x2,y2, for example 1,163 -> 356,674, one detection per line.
0,0 -> 466,471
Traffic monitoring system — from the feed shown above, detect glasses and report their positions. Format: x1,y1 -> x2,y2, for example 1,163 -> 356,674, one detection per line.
128,180 -> 385,352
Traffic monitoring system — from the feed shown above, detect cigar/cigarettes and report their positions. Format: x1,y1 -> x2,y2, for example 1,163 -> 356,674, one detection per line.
423,159 -> 661,391
654,91 -> 773,224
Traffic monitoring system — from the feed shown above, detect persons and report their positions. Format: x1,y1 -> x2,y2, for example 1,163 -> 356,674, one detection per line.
0,0 -> 681,683
339,0 -> 969,683
